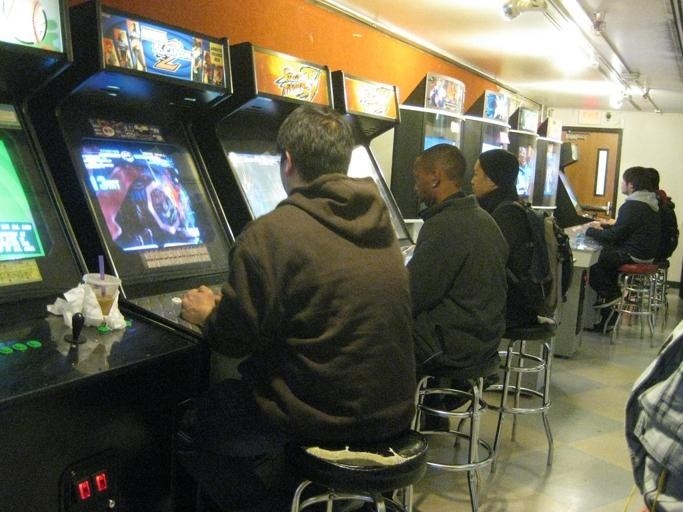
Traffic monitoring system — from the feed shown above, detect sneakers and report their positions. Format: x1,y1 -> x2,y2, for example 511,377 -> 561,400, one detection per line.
442,371 -> 501,412
593,309 -> 619,332
591,292 -> 622,309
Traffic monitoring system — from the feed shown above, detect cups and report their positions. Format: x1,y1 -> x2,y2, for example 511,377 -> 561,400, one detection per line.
69,329 -> 109,374
80,271 -> 121,323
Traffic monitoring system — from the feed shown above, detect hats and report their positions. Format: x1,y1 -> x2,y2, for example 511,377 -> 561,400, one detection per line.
478,149 -> 520,188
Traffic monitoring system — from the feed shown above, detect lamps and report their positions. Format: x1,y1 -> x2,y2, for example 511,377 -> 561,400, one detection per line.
500,0 -> 664,116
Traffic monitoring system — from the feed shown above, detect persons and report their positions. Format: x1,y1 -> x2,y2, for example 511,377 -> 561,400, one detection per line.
406,144 -> 509,433
167,102 -> 418,512
577,166 -> 662,309
443,149 -> 539,412
583,168 -> 679,333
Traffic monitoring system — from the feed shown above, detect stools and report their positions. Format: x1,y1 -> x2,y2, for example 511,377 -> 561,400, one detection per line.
602,259 -> 669,348
401,357 -> 502,512
448,314 -> 557,475
291,430 -> 429,512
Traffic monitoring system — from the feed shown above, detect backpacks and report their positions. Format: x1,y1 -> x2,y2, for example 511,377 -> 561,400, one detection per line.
491,195 -> 575,327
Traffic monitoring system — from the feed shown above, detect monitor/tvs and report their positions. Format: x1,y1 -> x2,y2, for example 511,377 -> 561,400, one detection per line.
543,152 -> 559,196
0,128 -> 47,265
424,136 -> 458,151
346,143 -> 410,243
514,143 -> 537,198
559,170 -> 579,208
80,136 -> 216,253
225,147 -> 289,222
481,143 -> 502,153
